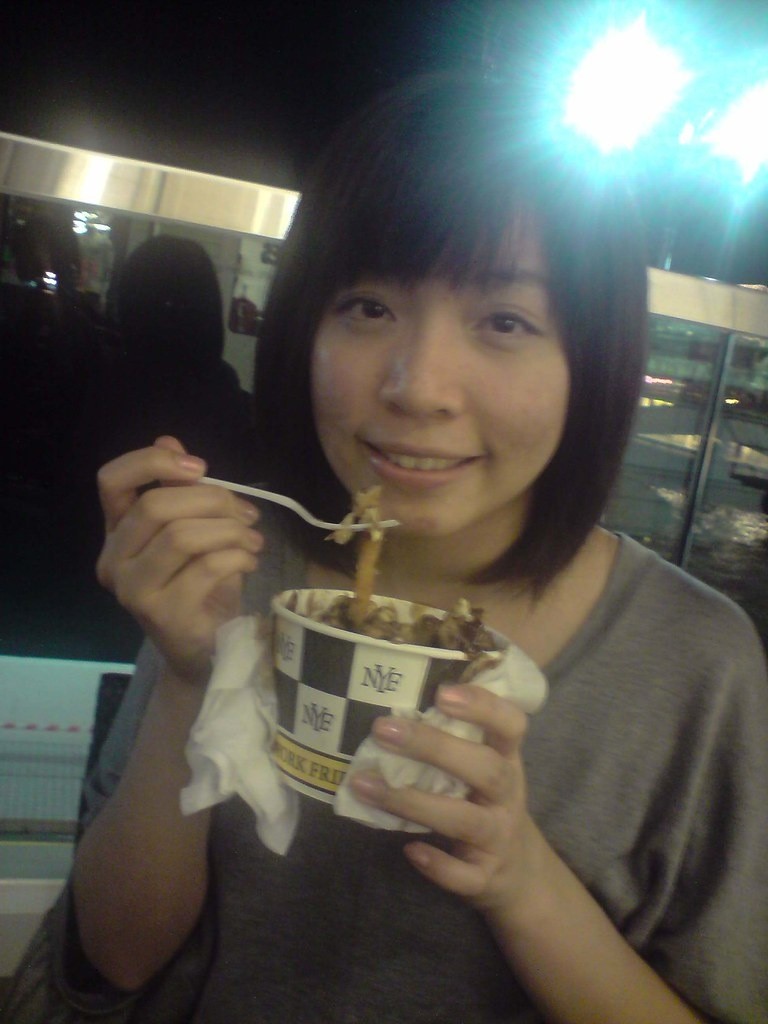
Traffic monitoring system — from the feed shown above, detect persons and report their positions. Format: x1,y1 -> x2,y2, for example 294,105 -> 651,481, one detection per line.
41,233 -> 242,661
51,70 -> 768,1024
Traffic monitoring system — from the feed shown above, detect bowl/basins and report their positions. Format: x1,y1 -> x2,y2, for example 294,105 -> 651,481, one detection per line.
267,589 -> 514,808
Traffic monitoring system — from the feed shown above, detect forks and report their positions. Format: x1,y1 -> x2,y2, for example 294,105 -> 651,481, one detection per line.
193,471 -> 402,530
